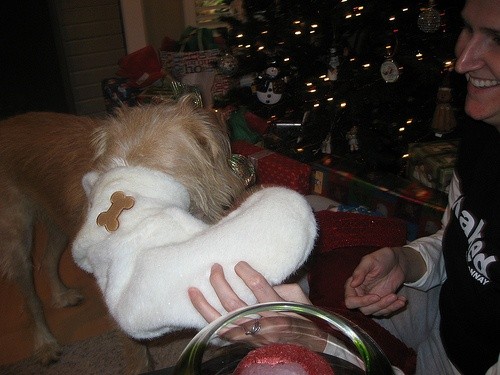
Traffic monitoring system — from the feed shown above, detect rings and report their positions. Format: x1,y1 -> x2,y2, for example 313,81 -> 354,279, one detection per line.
243,318 -> 263,337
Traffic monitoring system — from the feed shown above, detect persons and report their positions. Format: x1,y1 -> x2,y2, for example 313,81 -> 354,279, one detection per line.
189,1 -> 499,375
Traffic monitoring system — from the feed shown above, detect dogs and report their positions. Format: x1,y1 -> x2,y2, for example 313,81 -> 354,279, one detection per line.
1,93 -> 247,367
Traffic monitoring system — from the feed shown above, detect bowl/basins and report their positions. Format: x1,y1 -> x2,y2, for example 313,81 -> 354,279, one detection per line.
175,301 -> 396,375
